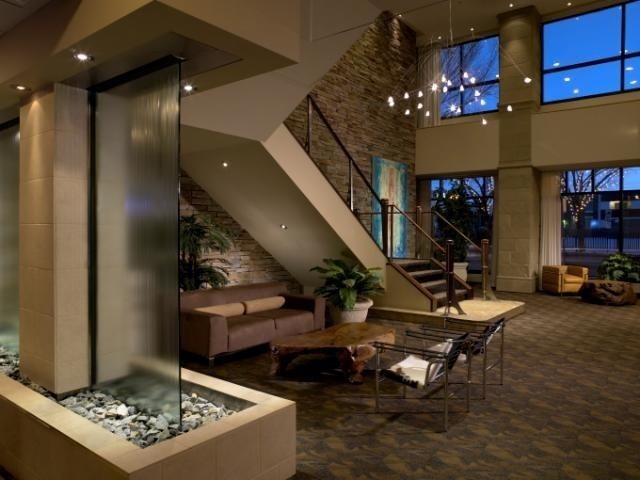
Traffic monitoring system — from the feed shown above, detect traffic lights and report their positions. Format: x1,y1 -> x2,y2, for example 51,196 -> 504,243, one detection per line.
241,296 -> 286,315
192,302 -> 245,318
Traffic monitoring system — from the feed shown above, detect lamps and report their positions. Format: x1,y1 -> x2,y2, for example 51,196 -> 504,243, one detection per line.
542,265 -> 589,298
368,330 -> 471,432
407,317 -> 505,399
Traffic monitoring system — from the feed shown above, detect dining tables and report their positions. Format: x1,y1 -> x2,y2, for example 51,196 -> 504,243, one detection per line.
433,188 -> 475,284
308,250 -> 384,327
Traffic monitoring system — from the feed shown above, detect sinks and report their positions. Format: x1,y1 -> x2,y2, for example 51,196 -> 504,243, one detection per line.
179,278 -> 328,368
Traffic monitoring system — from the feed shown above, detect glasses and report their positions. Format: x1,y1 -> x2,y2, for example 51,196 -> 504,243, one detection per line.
378,0 -> 532,125
